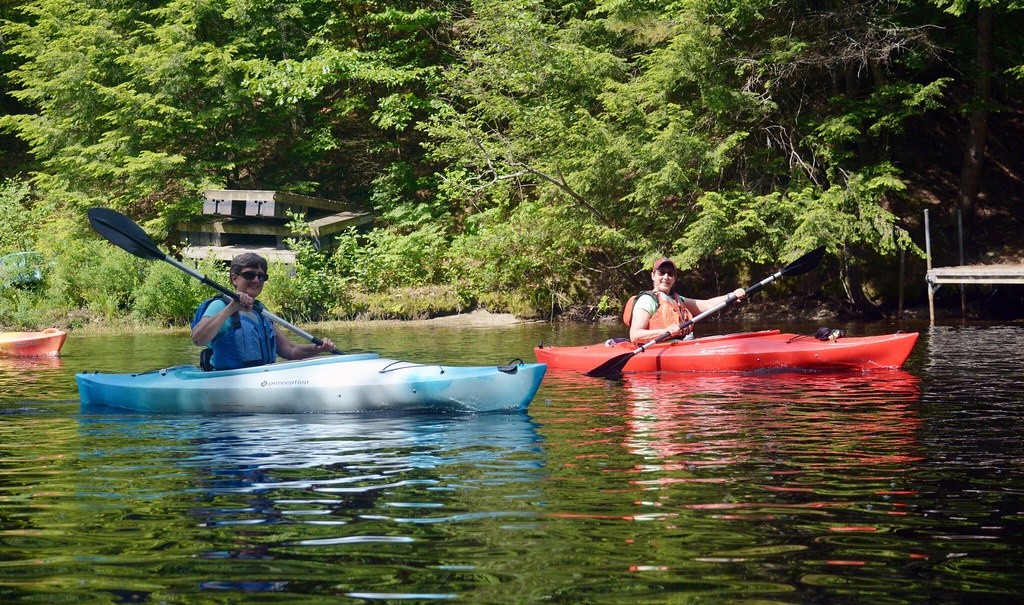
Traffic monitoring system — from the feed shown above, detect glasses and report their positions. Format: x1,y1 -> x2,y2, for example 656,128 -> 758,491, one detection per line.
236,271 -> 268,281
658,269 -> 676,277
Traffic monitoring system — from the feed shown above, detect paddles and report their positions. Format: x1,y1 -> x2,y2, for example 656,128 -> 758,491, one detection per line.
88,208 -> 348,355
585,244 -> 827,378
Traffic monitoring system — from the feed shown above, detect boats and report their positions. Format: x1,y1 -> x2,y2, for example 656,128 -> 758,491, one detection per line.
0,328 -> 66,356
74,350 -> 548,416
533,329 -> 920,374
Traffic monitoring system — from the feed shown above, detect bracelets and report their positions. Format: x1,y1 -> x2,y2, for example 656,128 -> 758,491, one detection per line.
728,293 -> 731,299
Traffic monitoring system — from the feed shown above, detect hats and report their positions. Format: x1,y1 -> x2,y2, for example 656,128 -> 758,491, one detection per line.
653,258 -> 678,274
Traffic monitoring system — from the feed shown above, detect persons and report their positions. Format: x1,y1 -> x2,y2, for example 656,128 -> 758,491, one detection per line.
629,258 -> 745,344
192,253 -> 336,371
